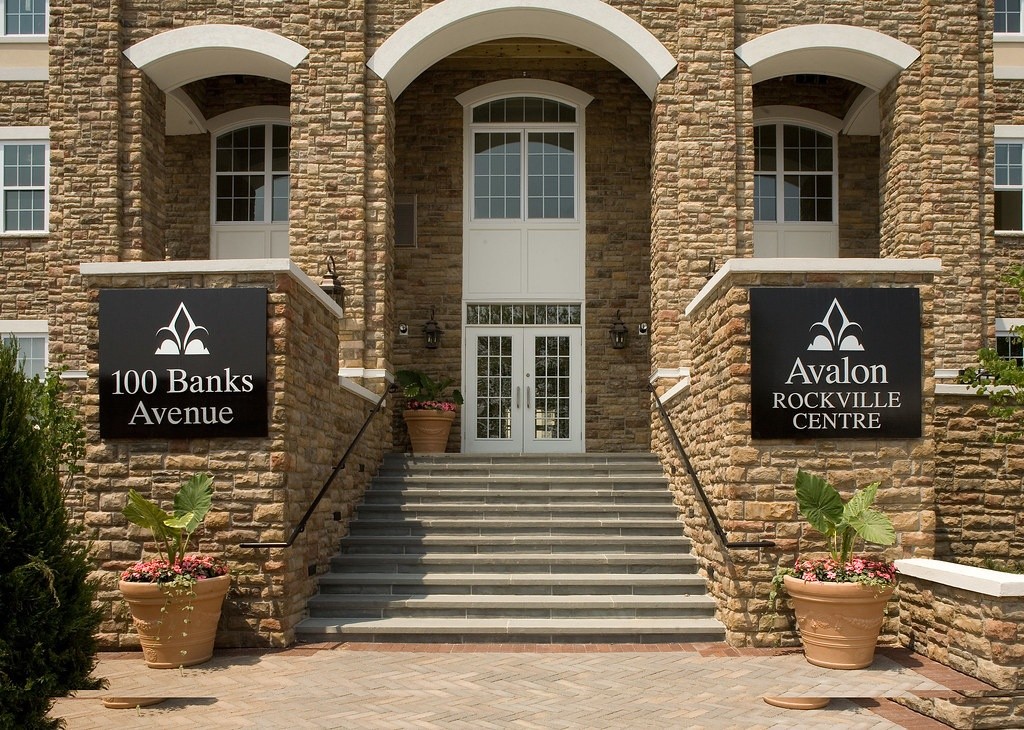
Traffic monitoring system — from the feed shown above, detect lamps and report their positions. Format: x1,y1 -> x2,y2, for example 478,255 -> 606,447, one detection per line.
420,306 -> 445,349
609,309 -> 628,349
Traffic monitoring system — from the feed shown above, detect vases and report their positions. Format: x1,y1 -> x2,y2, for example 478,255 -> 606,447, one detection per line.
783,575 -> 894,670
402,410 -> 455,453
120,573 -> 231,669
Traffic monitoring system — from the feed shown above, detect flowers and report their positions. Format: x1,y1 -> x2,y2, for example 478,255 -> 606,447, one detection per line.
119,471 -> 240,678
394,370 -> 464,411
766,467 -> 899,634
